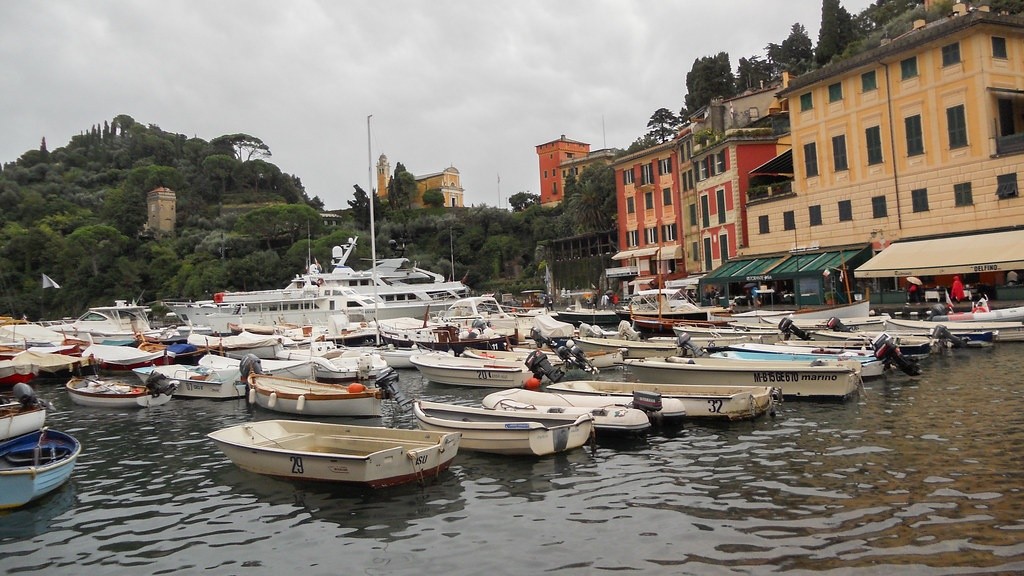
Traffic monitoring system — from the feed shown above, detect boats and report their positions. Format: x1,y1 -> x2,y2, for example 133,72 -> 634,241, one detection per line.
65,375 -> 181,409
413,395 -> 593,459
408,344 -> 626,389
210,417 -> 463,496
573,324 -> 782,368
228,298 -> 575,380
131,352 -> 316,399
482,387 -> 686,438
552,304 -> 743,325
0,297 -> 286,388
245,369 -> 383,417
162,234 -> 473,338
546,380 -> 782,422
615,307 -> 1024,398
0,393 -> 82,509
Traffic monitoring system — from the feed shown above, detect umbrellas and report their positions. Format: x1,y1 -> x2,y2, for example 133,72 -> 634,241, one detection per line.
744,283 -> 757,288
906,277 -> 922,286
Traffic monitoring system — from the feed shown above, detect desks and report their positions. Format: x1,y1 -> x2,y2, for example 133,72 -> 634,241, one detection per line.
924,291 -> 945,303
956,287 -> 978,299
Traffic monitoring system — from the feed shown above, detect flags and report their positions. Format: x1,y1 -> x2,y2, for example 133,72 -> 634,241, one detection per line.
43,275 -> 60,289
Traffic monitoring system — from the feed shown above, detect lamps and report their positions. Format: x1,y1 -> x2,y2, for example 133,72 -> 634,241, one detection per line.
871,228 -> 883,239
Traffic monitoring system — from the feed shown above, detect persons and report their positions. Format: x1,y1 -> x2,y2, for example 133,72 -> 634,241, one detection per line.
746,287 -> 761,309
705,287 -> 720,306
543,293 -> 549,311
601,293 -> 619,309
906,284 -> 920,305
950,276 -> 964,303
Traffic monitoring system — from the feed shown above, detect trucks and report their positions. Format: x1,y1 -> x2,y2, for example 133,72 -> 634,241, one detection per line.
627,288 -> 691,314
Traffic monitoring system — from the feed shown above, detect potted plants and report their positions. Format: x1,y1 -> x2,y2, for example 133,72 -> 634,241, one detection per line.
824,291 -> 836,304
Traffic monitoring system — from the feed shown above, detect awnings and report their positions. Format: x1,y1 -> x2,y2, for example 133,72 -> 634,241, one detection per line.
611,245 -> 682,261
853,230 -> 1024,277
